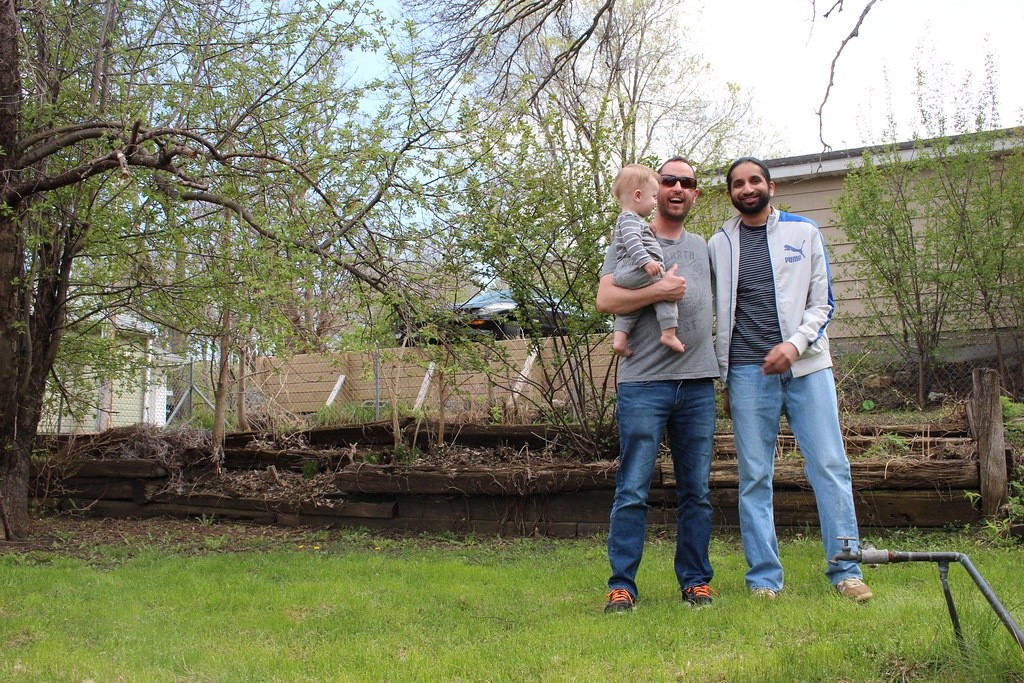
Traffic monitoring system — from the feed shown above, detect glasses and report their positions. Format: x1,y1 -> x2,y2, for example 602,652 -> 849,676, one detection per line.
661,175 -> 697,189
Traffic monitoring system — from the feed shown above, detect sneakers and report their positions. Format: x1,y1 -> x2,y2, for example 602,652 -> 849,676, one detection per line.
749,587 -> 776,602
605,588 -> 636,614
682,585 -> 719,610
836,577 -> 873,602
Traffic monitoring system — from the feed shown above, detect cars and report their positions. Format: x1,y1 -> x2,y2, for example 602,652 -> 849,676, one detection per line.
395,290 -> 614,348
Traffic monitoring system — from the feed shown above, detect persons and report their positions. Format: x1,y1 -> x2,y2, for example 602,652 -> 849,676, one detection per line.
707,157 -> 873,601
595,156 -> 720,619
612,164 -> 685,357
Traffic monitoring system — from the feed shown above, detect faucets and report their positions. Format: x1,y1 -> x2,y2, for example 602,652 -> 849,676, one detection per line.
827,536 -> 862,566
